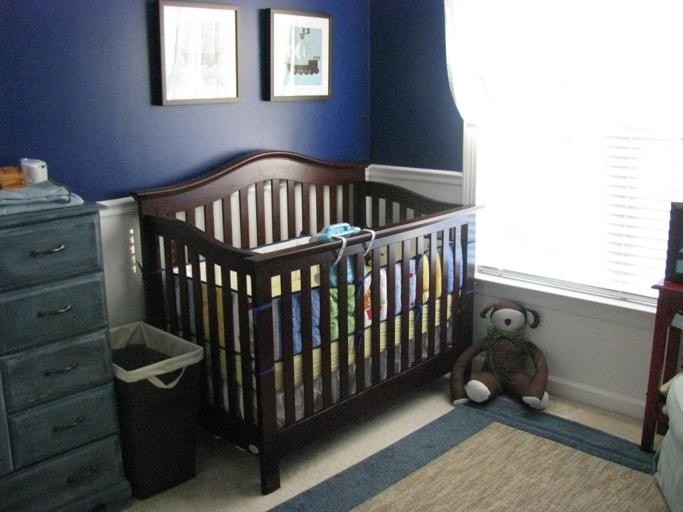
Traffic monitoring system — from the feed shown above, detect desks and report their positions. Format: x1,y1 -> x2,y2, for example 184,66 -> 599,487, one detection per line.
639,279 -> 683,449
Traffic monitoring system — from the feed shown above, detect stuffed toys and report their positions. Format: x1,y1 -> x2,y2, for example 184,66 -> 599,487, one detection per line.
447,298 -> 550,411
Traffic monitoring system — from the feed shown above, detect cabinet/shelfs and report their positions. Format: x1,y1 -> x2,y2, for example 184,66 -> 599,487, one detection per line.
0,189 -> 132,511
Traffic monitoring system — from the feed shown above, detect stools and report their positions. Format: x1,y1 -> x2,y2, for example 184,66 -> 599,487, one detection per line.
651,370 -> 682,512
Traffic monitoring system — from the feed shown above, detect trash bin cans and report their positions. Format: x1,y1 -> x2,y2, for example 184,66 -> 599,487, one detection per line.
109,321 -> 204,501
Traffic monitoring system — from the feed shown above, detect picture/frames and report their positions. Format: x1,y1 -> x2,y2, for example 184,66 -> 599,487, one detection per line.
263,6 -> 333,104
152,0 -> 242,107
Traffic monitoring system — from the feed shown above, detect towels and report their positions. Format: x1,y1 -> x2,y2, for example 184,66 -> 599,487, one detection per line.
0,177 -> 83,214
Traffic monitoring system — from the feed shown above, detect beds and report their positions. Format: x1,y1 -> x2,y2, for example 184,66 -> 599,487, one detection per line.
132,148 -> 486,498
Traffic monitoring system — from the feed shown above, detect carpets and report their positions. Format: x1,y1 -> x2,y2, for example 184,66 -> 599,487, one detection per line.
265,395 -> 671,512
